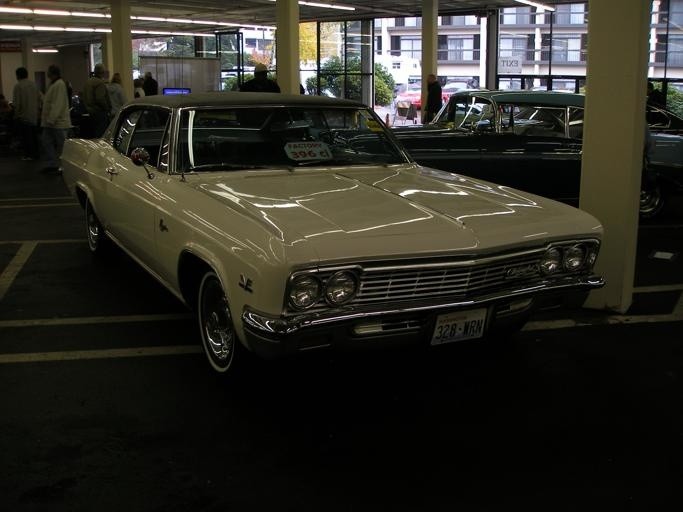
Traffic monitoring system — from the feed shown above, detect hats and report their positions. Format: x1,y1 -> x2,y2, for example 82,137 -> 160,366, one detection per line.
254,62 -> 270,73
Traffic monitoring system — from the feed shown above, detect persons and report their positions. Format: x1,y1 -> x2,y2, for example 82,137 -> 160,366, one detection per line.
646,82 -> 666,109
38,64 -> 71,176
133,77 -> 145,100
105,72 -> 128,121
84,62 -> 112,139
9,66 -> 46,161
142,71 -> 158,97
425,74 -> 442,124
238,63 -> 281,94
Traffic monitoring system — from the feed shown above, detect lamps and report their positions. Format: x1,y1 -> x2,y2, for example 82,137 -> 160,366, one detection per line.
0,3 -> 112,21
514,1 -> 553,14
298,1 -> 356,14
131,26 -> 216,39
130,13 -> 277,30
0,21 -> 112,34
